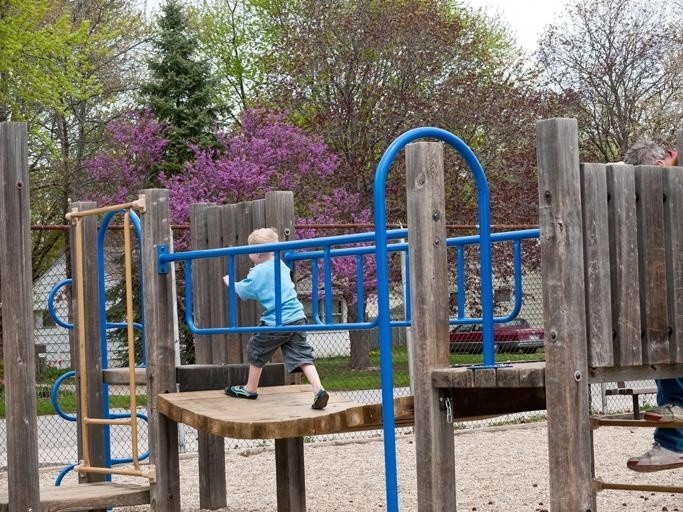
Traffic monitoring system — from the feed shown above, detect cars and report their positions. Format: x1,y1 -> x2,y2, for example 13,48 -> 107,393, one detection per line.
450,317 -> 543,355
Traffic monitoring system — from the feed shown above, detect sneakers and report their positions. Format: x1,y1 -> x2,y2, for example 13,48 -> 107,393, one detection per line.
627,444 -> 683,472
225,386 -> 258,399
644,402 -> 683,424
312,389 -> 329,409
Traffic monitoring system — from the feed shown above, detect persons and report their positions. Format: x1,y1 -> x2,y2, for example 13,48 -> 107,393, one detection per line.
618,137 -> 682,471
221,227 -> 328,409
